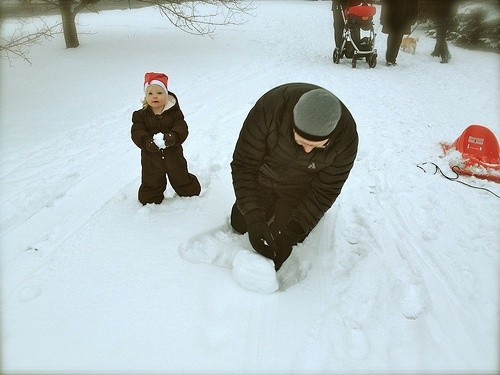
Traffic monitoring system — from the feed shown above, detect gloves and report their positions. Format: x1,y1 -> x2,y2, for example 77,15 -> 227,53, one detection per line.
164,132 -> 177,145
145,138 -> 159,152
244,209 -> 302,273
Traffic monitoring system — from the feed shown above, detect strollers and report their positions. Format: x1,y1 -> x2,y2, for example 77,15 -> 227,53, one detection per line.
331,0 -> 378,69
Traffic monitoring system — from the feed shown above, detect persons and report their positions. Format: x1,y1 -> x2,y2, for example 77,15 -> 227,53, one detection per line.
349,0 -> 453,67
230,83 -> 358,271
131,72 -> 202,205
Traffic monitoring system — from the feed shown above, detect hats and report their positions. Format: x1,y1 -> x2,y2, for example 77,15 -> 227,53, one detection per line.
144,72 -> 168,96
292,88 -> 342,141
